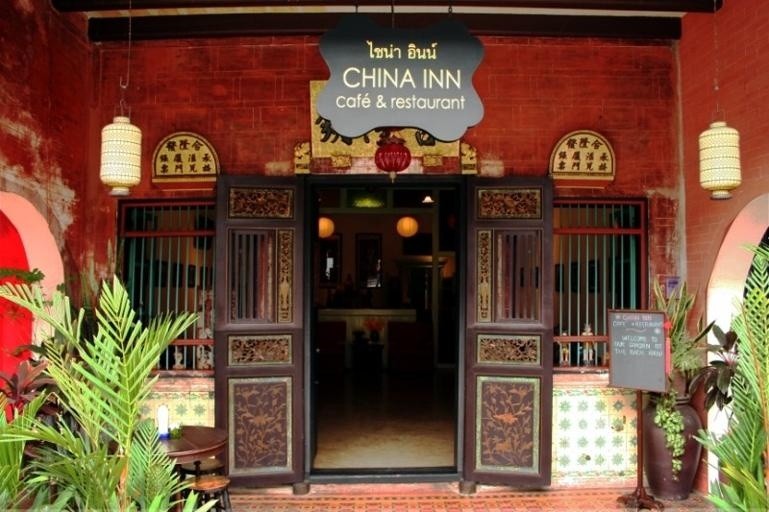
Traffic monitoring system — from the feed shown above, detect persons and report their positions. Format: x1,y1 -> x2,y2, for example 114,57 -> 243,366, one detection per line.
555,329 -> 573,367
581,322 -> 598,364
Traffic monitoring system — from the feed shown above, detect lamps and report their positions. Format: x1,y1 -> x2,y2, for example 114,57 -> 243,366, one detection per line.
318,217 -> 335,239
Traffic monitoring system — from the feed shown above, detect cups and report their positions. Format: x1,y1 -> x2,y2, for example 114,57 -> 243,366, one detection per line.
170,423 -> 183,439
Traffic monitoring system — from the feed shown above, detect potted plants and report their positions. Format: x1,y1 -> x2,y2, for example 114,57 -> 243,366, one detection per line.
689,323 -> 749,485
648,272 -> 716,482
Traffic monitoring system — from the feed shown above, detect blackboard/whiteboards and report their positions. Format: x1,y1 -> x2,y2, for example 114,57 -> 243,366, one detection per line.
607,309 -> 669,393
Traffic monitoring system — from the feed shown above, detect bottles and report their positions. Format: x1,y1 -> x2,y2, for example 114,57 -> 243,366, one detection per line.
158,403 -> 169,440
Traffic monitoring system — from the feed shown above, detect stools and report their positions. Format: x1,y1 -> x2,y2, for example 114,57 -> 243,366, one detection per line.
180,458 -> 226,503
182,474 -> 231,512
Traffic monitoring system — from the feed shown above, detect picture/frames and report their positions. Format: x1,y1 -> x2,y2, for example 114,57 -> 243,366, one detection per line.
520,258 -> 603,294
354,232 -> 384,289
144,259 -> 214,292
318,232 -> 343,288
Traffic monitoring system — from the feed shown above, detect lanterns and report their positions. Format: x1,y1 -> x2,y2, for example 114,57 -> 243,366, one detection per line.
316,218 -> 335,239
100,116 -> 141,196
698,121 -> 742,201
375,136 -> 411,182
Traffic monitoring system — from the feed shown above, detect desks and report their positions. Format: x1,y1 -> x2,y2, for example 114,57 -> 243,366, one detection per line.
108,425 -> 232,512
